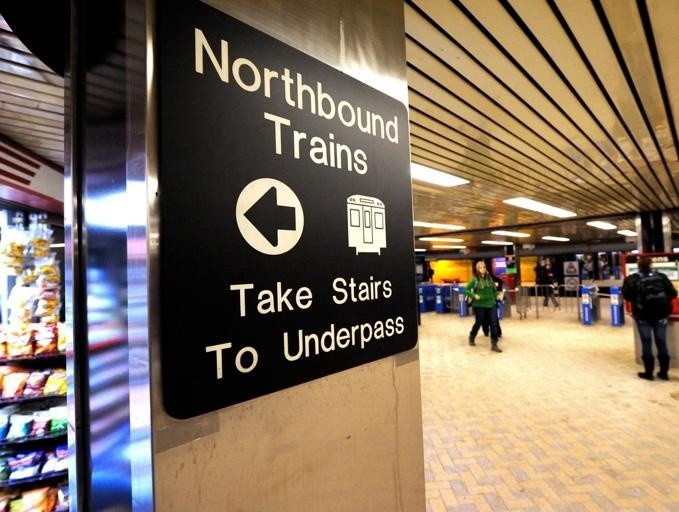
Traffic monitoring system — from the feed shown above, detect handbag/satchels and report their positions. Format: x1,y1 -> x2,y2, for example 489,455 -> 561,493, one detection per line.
465,295 -> 474,307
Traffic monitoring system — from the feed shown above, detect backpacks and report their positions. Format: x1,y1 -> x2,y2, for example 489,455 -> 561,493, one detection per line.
635,274 -> 673,322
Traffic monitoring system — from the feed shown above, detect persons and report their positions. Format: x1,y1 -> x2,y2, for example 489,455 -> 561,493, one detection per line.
481,271 -> 504,338
621,254 -> 678,381
543,271 -> 559,307
463,261 -> 503,353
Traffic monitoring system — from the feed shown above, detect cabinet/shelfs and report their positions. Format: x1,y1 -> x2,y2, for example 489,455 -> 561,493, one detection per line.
0,180 -> 69,512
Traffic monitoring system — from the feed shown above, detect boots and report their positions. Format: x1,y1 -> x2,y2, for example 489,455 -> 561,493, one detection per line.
491,338 -> 501,352
658,356 -> 670,380
469,335 -> 475,346
639,358 -> 655,379
